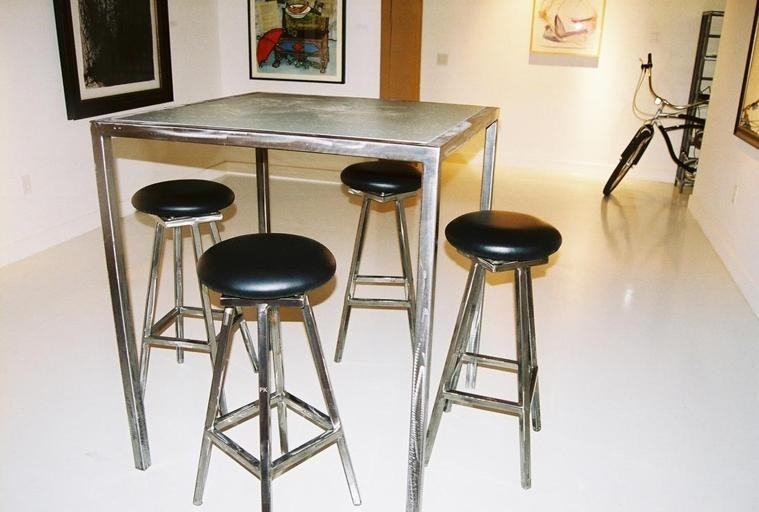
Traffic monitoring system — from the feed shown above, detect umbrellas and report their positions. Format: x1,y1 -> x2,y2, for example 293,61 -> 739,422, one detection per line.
257,28 -> 292,67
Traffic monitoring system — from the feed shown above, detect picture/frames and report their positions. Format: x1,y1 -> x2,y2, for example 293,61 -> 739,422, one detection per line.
733,0 -> 759,150
52,0 -> 174,121
529,0 -> 606,59
247,0 -> 346,84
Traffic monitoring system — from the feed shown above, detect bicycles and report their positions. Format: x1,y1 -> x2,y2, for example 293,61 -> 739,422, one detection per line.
601,49 -> 709,200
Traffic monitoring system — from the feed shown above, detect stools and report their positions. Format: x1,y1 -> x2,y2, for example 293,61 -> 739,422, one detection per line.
425,211 -> 562,489
131,178 -> 258,418
333,161 -> 422,363
193,233 -> 361,512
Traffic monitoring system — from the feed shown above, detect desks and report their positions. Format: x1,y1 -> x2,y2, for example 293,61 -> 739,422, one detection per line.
88,92 -> 501,512
